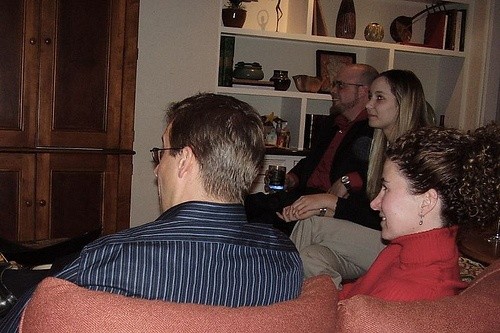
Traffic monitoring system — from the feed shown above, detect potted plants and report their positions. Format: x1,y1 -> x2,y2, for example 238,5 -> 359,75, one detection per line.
222,0 -> 249,28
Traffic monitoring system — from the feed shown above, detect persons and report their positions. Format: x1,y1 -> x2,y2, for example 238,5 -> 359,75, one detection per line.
0,91 -> 304,333
244,63 -> 379,238
338,119 -> 500,303
276,68 -> 436,292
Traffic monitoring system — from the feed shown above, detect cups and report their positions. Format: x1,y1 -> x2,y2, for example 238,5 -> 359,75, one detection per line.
268,166 -> 286,189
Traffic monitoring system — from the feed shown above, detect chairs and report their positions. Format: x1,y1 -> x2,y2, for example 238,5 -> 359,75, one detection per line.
336,264 -> 500,332
16,273 -> 337,332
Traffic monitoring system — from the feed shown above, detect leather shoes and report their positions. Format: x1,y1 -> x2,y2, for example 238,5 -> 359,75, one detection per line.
0,251 -> 23,318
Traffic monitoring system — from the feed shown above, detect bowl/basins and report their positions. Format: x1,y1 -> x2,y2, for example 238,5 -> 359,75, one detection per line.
292,75 -> 325,93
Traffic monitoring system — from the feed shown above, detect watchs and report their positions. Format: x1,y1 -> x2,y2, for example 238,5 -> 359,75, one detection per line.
341,175 -> 352,190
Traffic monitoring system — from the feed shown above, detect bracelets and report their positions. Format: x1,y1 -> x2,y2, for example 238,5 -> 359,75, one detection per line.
317,208 -> 326,216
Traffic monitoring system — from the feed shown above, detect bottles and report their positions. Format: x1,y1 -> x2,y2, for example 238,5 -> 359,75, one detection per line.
270,70 -> 291,90
335,0 -> 357,39
278,123 -> 290,148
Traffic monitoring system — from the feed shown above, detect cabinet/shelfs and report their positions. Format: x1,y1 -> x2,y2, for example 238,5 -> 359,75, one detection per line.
214,0 -> 494,194
1,152 -> 133,271
0,0 -> 140,154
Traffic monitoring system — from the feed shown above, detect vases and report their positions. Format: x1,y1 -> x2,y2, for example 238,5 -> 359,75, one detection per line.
336,0 -> 356,38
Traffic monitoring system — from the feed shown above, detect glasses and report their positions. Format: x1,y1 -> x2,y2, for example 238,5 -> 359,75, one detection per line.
150,146 -> 183,164
331,80 -> 363,89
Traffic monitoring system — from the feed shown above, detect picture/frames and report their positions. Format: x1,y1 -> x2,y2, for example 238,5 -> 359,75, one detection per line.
315,49 -> 356,94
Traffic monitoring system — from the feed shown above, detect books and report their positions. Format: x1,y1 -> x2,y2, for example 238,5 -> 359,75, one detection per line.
312,0 -> 328,36
424,9 -> 467,52
218,35 -> 236,87
